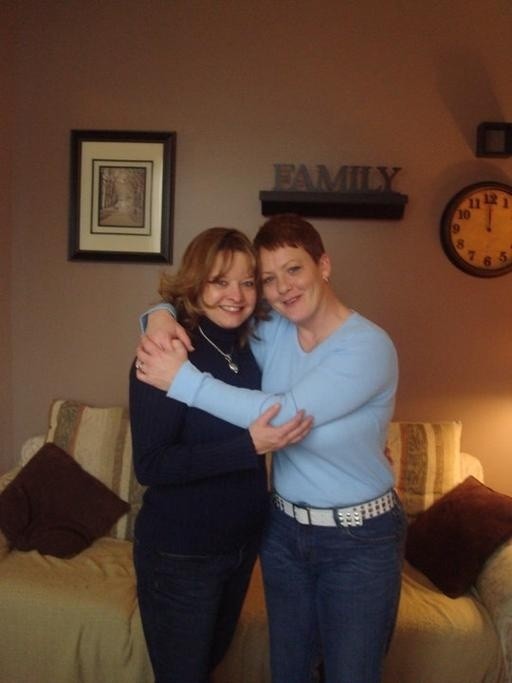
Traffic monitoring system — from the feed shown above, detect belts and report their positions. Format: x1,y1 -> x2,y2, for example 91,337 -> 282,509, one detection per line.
276,492 -> 393,527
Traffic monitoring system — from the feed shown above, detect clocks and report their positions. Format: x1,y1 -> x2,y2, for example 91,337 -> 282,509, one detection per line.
439,181 -> 512,278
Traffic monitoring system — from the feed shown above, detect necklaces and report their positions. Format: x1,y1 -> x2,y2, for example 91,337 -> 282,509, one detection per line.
197,322 -> 241,376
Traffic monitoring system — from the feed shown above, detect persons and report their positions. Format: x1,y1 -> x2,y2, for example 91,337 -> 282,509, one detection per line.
135,214 -> 407,680
130,228 -> 314,681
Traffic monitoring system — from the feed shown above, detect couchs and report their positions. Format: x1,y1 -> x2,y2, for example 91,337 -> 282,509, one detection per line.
1,398 -> 512,682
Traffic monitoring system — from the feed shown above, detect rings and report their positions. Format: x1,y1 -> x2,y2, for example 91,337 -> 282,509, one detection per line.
134,360 -> 142,371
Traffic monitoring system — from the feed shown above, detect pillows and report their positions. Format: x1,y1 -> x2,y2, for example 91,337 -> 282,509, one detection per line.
1,442 -> 132,560
404,475 -> 512,599
44,398 -> 144,541
385,420 -> 463,528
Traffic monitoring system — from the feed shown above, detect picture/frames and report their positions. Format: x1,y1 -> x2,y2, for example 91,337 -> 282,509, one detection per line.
69,129 -> 177,265
